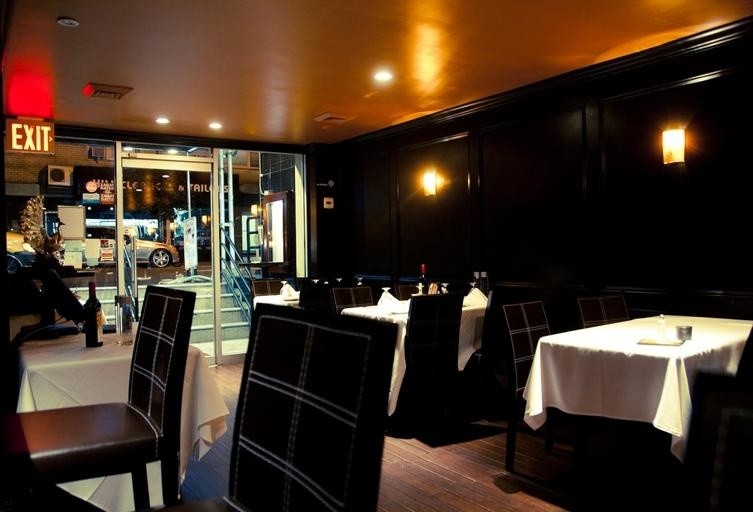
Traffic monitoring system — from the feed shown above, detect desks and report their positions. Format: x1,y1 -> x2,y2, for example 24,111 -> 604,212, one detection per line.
533,315 -> 753,510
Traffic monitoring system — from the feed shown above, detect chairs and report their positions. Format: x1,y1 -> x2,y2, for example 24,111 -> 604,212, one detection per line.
498,300 -> 560,474
250,280 -> 538,447
223,301 -> 396,512
574,294 -> 631,329
15,284 -> 198,512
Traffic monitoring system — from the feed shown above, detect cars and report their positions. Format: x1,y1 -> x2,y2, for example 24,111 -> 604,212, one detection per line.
50,226 -> 180,268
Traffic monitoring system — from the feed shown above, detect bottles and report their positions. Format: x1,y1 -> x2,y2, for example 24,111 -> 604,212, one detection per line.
114,294 -> 133,346
84,280 -> 104,347
472,271 -> 490,297
416,263 -> 429,295
